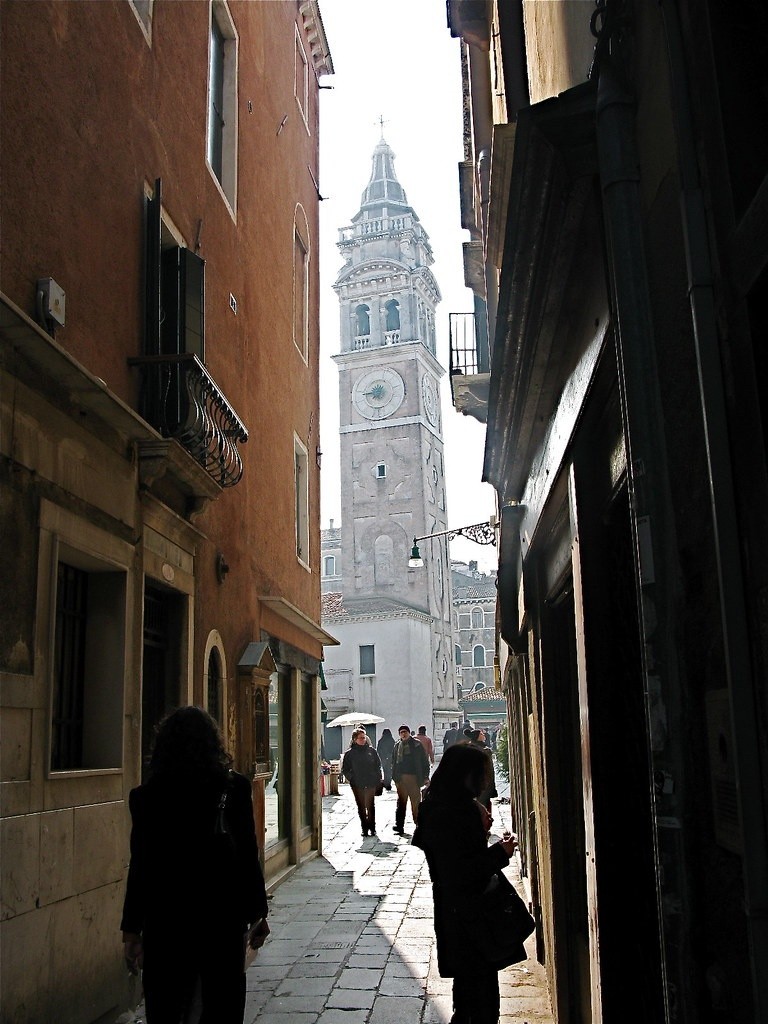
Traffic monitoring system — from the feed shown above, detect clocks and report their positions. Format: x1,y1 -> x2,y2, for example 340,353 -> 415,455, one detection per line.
423,372 -> 440,428
352,368 -> 405,420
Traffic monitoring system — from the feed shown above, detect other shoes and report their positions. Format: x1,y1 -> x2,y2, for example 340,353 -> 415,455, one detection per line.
392,826 -> 404,834
361,829 -> 376,837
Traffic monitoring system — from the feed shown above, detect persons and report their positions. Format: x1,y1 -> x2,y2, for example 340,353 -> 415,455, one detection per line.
117,705 -> 270,1024
390,723 -> 429,837
376,727 -> 397,792
442,717 -> 499,830
411,724 -> 434,787
411,742 -> 538,1024
340,728 -> 384,838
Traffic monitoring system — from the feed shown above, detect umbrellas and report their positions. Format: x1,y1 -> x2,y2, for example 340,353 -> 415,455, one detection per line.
326,710 -> 386,731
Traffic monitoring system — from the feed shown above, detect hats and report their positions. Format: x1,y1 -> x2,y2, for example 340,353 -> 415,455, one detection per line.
399,725 -> 411,736
484,728 -> 489,733
463,728 -> 481,743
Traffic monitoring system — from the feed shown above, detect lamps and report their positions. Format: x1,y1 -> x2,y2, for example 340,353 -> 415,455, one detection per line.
409,544 -> 425,569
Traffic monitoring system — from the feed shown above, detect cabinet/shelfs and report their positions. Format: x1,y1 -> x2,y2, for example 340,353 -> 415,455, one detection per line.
331,774 -> 339,795
320,774 -> 330,796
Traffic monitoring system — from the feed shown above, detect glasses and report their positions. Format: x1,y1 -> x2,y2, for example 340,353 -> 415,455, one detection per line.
357,737 -> 366,740
400,730 -> 408,734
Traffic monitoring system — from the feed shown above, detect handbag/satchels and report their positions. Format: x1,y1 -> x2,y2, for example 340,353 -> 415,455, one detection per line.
460,893 -> 537,965
208,771 -> 257,925
